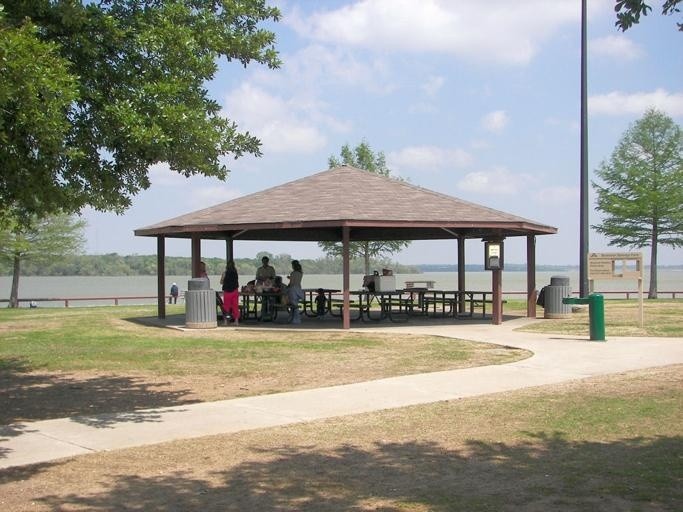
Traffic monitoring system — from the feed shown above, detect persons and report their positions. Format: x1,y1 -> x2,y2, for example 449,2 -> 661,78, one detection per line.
287,260 -> 303,325
169,283 -> 178,304
220,261 -> 241,326
200,262 -> 224,316
315,288 -> 326,319
266,276 -> 286,320
256,257 -> 276,321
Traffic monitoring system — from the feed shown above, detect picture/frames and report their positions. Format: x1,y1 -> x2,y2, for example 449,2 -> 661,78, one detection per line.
485,241 -> 504,270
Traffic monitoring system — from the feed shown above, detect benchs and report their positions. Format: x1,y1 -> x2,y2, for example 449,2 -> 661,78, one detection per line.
405,282 -> 437,289
216,289 -> 507,324
